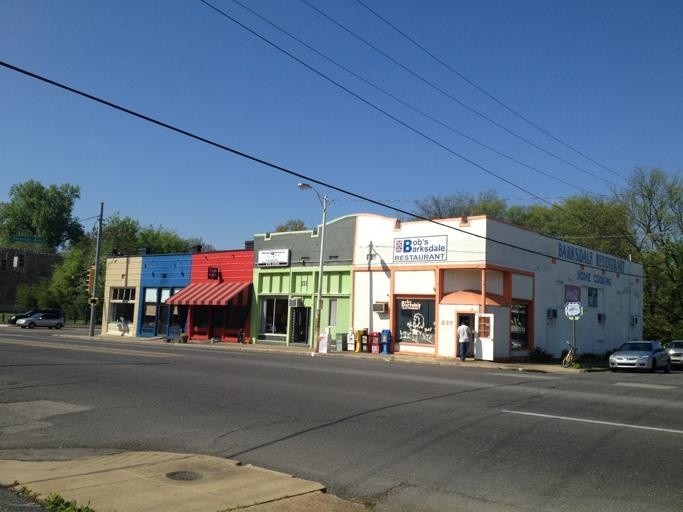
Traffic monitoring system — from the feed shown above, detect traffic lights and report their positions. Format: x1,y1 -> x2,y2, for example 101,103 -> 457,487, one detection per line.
85,269 -> 99,305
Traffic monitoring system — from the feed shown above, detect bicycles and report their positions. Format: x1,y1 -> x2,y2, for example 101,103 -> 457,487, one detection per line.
562,340 -> 580,367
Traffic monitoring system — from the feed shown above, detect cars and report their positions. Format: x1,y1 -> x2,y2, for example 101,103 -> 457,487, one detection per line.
8,309 -> 65,329
609,340 -> 683,373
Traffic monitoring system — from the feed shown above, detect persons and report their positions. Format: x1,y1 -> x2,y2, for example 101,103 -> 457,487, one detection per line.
457,318 -> 472,361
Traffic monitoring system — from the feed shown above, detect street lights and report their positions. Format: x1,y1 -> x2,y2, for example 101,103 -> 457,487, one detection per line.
298,183 -> 327,352
